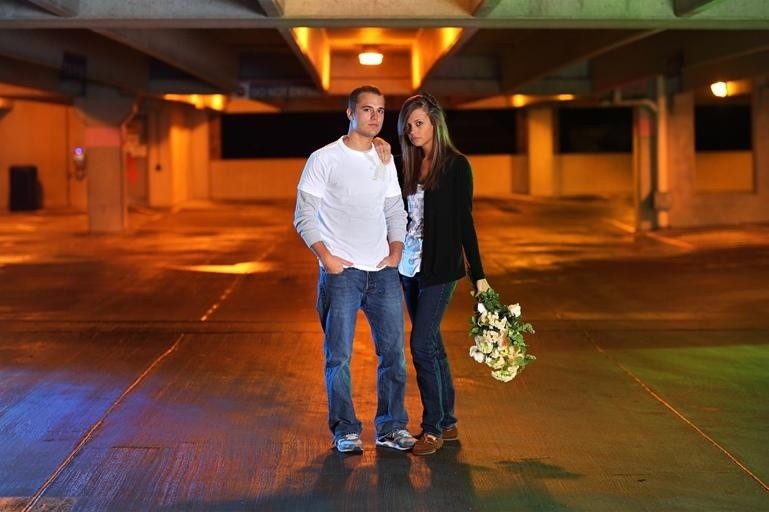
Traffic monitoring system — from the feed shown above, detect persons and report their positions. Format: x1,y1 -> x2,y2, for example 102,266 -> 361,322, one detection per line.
291,84 -> 417,455
372,91 -> 492,457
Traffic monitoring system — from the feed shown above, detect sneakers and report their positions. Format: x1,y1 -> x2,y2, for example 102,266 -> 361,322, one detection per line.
412,431 -> 443,456
420,421 -> 458,442
375,429 -> 416,451
334,433 -> 364,454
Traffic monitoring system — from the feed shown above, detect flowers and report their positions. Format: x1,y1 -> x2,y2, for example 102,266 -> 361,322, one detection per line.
464,263 -> 539,385
